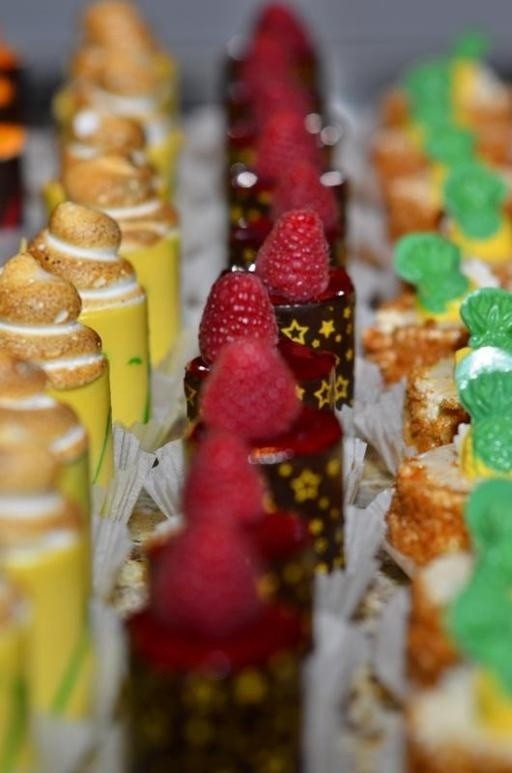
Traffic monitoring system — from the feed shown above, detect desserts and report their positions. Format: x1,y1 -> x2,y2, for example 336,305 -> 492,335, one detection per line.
0,1 -> 512,773
